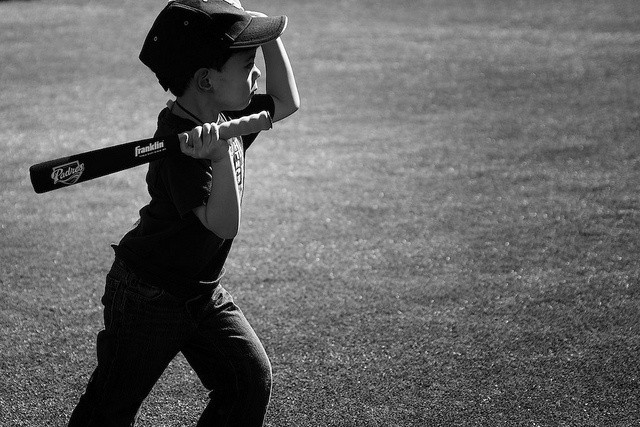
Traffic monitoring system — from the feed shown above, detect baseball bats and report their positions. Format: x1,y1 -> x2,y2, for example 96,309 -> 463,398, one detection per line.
30,111 -> 272,194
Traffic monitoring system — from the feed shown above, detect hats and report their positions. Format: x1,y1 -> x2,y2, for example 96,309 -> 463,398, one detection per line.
138,0 -> 289,91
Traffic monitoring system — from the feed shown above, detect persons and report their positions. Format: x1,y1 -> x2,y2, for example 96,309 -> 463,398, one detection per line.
67,1 -> 301,425
